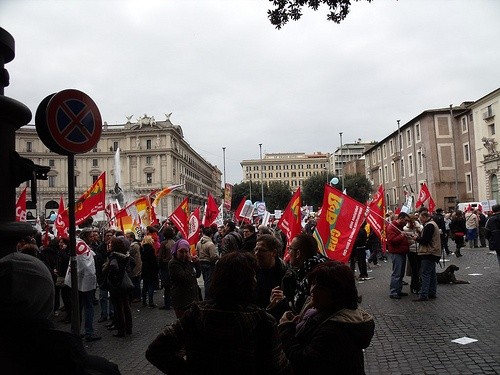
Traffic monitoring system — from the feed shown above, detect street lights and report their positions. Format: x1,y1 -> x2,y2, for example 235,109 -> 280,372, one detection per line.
259,143 -> 266,202
339,132 -> 348,191
222,146 -> 230,188
397,119 -> 405,199
245,173 -> 253,200
449,104 -> 460,211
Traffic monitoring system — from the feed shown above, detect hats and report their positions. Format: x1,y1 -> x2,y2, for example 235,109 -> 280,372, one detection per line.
171,238 -> 191,256
0,252 -> 55,321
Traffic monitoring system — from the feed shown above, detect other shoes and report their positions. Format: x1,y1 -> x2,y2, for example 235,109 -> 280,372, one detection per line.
389,293 -> 401,299
354,258 -> 389,280
112,328 -> 132,338
98,318 -> 107,323
104,321 -> 118,331
447,240 -> 486,258
123,286 -> 171,311
398,292 -> 408,296
412,292 -> 437,302
81,334 -> 102,343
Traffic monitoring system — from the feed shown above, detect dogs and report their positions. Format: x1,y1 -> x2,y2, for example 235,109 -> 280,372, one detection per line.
435,264 -> 471,284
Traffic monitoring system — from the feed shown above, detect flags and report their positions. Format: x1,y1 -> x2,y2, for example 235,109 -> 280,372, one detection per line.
416,183 -> 436,216
467,205 -> 471,213
277,188 -> 305,263
401,187 -> 416,213
364,185 -> 386,256
16,190 -> 70,245
54,171 -> 105,238
478,204 -> 482,212
169,195 -> 224,246
234,196 -> 251,224
115,179 -> 183,235
312,184 -> 373,265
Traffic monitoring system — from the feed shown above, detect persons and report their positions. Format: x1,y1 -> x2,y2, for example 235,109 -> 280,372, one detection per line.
109,187 -> 126,208
0,204 -> 500,375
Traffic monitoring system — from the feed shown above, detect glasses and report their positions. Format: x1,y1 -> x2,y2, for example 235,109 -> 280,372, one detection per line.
289,246 -> 297,252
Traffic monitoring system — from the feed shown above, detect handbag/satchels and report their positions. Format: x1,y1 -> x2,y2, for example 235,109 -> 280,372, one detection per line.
55,276 -> 66,287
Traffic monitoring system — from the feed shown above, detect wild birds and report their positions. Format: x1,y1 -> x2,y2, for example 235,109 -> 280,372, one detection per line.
125,115 -> 133,120
164,112 -> 173,118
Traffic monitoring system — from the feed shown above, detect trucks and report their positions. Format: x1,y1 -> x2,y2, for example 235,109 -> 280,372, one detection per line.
302,206 -> 315,217
482,199 -> 497,214
457,203 -> 483,214
270,209 -> 284,219
